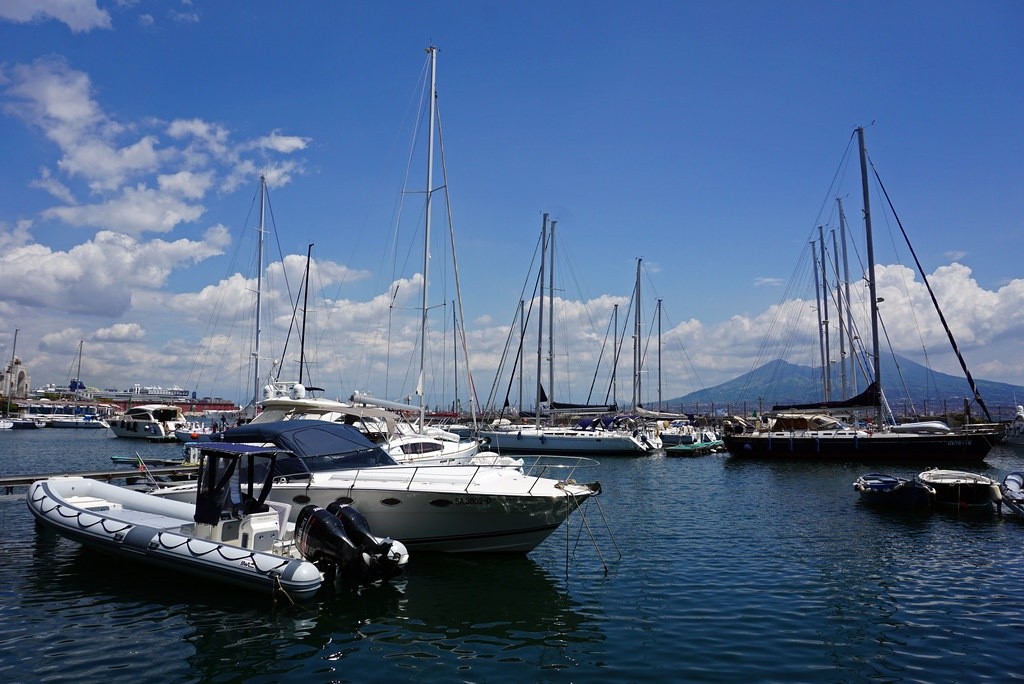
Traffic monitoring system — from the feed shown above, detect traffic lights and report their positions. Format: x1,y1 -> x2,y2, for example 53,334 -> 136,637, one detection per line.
0,44 -> 1004,469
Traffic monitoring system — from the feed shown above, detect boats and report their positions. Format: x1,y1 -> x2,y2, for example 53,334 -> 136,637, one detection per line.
25,441 -> 410,600
145,420 -> 604,558
918,465 -> 1005,523
853,470 -> 937,506
1001,471 -> 1024,521
